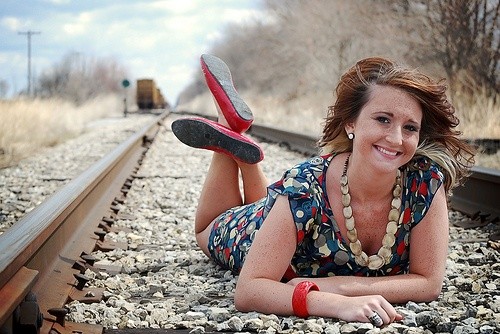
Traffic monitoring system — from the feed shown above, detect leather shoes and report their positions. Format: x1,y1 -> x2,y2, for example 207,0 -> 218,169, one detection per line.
199,53 -> 254,137
170,115 -> 264,168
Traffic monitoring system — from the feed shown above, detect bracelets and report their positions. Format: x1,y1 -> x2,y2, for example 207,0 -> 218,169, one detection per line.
292,280 -> 319,318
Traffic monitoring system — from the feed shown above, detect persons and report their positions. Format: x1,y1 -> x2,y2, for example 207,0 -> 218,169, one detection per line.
170,52 -> 478,326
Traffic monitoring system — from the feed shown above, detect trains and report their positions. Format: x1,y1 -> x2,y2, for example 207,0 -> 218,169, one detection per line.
136,79 -> 168,111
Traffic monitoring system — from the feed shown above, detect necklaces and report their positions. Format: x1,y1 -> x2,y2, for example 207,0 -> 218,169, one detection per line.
340,152 -> 406,270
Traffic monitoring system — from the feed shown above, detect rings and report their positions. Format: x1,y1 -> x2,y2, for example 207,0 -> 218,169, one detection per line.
368,313 -> 382,325
